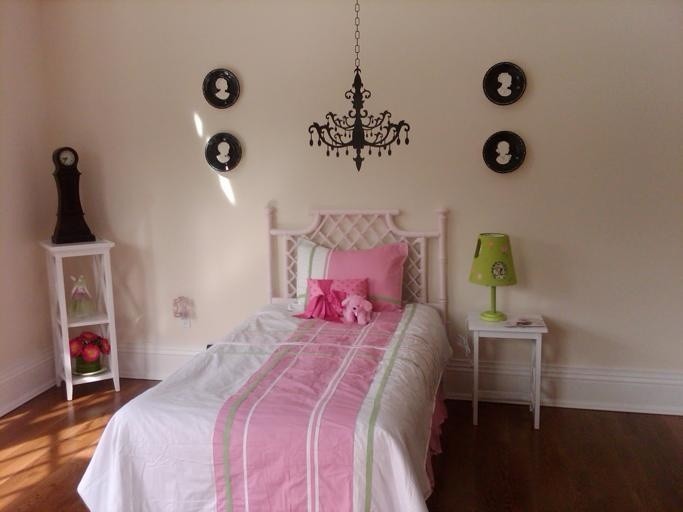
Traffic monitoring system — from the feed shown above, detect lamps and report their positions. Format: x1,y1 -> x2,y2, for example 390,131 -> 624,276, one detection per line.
308,0 -> 410,171
468,232 -> 517,322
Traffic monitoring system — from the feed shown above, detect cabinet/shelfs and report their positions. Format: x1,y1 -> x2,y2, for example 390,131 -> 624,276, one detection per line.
38,234 -> 121,401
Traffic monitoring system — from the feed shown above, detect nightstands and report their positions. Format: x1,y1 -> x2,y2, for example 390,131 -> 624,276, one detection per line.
464,312 -> 549,431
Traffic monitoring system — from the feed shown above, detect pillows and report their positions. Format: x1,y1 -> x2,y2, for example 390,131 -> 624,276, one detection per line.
296,237 -> 409,313
292,278 -> 370,323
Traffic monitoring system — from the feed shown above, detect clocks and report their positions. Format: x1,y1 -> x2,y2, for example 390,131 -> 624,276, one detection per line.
51,146 -> 96,243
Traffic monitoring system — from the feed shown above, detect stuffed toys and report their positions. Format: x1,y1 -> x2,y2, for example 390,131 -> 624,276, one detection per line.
340,295 -> 373,324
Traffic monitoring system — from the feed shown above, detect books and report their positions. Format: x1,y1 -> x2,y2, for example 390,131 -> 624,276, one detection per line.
503,317 -> 546,328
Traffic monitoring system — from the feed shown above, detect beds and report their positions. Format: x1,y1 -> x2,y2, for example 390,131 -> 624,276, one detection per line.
76,207 -> 455,512
